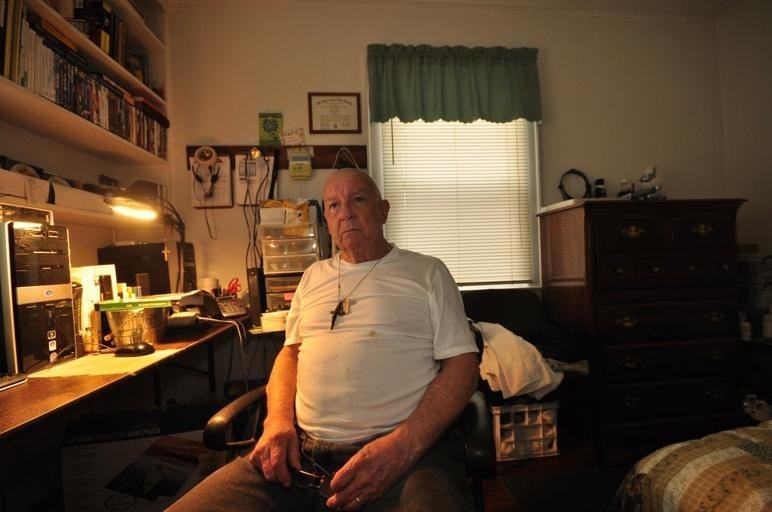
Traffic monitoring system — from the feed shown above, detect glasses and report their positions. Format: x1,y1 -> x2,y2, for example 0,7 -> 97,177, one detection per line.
292,436 -> 335,498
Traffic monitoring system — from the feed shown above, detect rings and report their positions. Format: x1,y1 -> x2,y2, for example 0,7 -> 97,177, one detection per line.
356,496 -> 361,505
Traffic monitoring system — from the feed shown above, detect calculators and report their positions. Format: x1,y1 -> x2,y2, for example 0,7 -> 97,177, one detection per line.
216,296 -> 246,317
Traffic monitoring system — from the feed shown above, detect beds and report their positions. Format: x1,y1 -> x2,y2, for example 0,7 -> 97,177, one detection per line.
613,419 -> 772,512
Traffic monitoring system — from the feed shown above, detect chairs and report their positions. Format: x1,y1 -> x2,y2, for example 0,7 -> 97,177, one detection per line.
203,317 -> 498,511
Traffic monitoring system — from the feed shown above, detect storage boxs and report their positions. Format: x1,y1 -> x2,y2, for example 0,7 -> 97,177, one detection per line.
735,253 -> 772,337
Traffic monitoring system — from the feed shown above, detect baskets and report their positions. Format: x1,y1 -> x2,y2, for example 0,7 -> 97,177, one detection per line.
492,401 -> 560,462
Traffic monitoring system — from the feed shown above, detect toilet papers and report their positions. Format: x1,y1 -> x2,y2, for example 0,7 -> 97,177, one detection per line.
201,278 -> 218,296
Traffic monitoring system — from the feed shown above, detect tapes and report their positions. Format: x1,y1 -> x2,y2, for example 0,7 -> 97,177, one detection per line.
195,147 -> 217,165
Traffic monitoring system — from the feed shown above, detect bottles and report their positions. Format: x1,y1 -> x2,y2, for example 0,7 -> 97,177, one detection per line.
126,286 -> 132,297
137,286 -> 142,296
117,282 -> 127,299
132,287 -> 138,296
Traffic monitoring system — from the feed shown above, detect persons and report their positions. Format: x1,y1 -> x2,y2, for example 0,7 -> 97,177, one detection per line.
160,165 -> 482,510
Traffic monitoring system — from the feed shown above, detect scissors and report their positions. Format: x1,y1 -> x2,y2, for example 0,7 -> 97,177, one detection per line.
228,278 -> 241,293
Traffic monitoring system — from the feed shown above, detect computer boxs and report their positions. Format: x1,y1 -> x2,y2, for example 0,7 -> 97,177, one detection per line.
1,222 -> 78,377
97,242 -> 197,295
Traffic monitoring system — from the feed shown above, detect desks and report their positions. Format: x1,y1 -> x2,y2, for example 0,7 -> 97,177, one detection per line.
1,304 -> 251,440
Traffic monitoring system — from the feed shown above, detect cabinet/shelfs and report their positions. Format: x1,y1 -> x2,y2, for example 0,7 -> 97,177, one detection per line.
0,0 -> 171,162
536,198 -> 751,454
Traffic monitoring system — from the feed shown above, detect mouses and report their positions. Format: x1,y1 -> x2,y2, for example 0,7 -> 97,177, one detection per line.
112,342 -> 155,356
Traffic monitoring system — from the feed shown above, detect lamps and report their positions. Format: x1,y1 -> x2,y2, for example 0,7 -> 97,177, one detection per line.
103,180 -> 186,311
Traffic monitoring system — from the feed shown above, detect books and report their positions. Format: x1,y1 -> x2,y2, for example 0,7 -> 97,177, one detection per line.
0,0 -> 171,163
118,282 -> 142,299
94,288 -> 205,312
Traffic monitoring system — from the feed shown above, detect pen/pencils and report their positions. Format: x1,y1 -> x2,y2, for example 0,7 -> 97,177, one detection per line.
212,288 -> 228,297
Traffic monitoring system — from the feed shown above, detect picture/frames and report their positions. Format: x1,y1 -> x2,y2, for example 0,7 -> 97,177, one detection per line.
307,91 -> 361,135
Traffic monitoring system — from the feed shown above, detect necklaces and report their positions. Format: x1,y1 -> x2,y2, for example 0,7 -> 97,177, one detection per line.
329,244 -> 391,331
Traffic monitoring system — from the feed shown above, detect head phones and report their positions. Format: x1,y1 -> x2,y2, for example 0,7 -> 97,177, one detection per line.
191,159 -> 222,183
